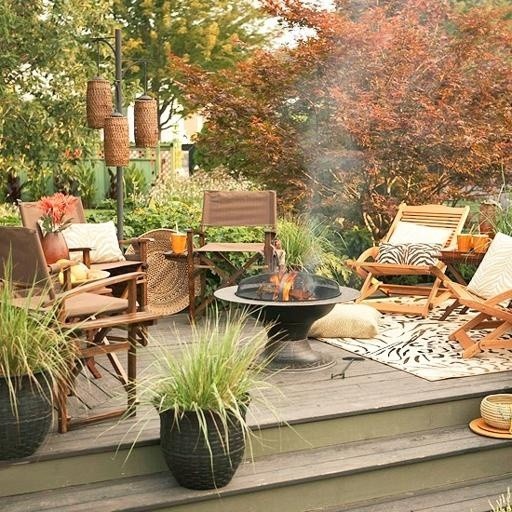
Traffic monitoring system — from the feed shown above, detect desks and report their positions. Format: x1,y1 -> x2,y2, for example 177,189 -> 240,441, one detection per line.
431,254 -> 487,322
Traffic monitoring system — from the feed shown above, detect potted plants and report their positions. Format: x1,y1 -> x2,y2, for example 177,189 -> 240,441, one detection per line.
0,237 -> 110,463
92,292 -> 312,489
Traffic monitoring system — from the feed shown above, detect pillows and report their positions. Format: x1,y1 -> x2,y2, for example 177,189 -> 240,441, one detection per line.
374,241 -> 443,269
308,302 -> 383,340
466,230 -> 512,306
388,219 -> 453,250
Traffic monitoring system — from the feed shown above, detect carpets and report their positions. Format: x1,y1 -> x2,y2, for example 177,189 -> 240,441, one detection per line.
314,288 -> 512,383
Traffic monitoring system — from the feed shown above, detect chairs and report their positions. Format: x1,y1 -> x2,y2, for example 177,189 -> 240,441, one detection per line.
345,200 -> 472,315
437,232 -> 512,360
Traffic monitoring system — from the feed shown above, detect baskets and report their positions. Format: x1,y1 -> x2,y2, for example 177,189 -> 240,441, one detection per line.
125,228 -> 200,314
480,393 -> 512,429
